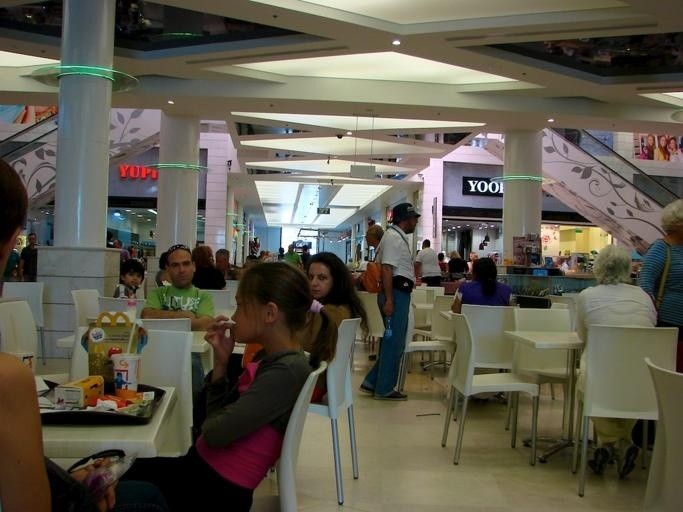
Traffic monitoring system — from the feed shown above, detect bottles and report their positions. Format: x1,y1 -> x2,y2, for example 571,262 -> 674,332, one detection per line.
125,301 -> 136,322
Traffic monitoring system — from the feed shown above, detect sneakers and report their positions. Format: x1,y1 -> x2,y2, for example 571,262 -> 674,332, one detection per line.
612,439 -> 639,479
587,443 -> 612,474
358,384 -> 407,401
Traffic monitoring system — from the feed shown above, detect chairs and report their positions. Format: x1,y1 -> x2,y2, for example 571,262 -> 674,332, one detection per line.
353,283 -> 579,467
253,318 -> 361,511
1,277 -> 245,510
572,324 -> 677,510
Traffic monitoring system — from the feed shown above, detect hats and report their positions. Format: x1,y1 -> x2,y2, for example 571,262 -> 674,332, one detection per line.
389,203 -> 421,223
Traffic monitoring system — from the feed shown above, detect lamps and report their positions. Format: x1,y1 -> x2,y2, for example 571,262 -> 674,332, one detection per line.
479,230 -> 490,250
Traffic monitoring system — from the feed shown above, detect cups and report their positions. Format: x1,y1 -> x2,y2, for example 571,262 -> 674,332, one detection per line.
10,353 -> 36,376
110,352 -> 144,400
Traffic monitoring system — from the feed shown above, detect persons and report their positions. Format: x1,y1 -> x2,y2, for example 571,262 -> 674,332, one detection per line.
413,238 -> 514,314
357,203 -> 421,400
5,234 -> 38,282
141,243 -> 216,393
651,135 -> 670,160
574,245 -> 658,477
633,199 -> 681,375
639,133 -> 655,159
114,259 -> 314,512
0,155 -> 116,512
113,260 -> 144,299
106,234 -> 139,262
299,252 -> 367,403
366,218 -> 384,245
155,246 -> 240,290
667,137 -> 682,161
246,245 -> 312,273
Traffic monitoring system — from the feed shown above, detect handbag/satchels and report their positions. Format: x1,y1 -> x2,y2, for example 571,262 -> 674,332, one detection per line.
393,275 -> 414,294
361,261 -> 384,293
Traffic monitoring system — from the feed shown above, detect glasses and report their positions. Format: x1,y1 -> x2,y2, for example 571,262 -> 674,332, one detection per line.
67,449 -> 127,474
167,244 -> 193,256
